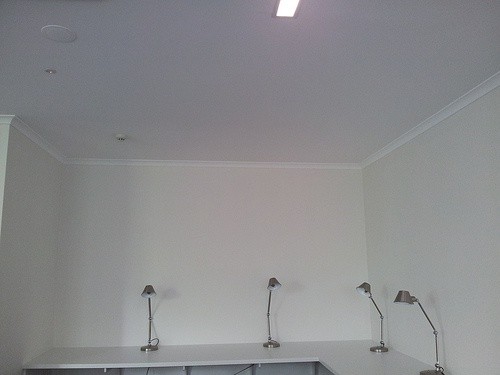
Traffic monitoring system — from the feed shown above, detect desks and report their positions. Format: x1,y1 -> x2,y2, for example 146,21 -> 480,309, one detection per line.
23,341 -> 444,375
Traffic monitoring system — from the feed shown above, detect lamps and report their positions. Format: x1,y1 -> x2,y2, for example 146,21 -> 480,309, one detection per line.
393,290 -> 445,375
263,277 -> 282,347
356,282 -> 388,352
139,285 -> 159,351
